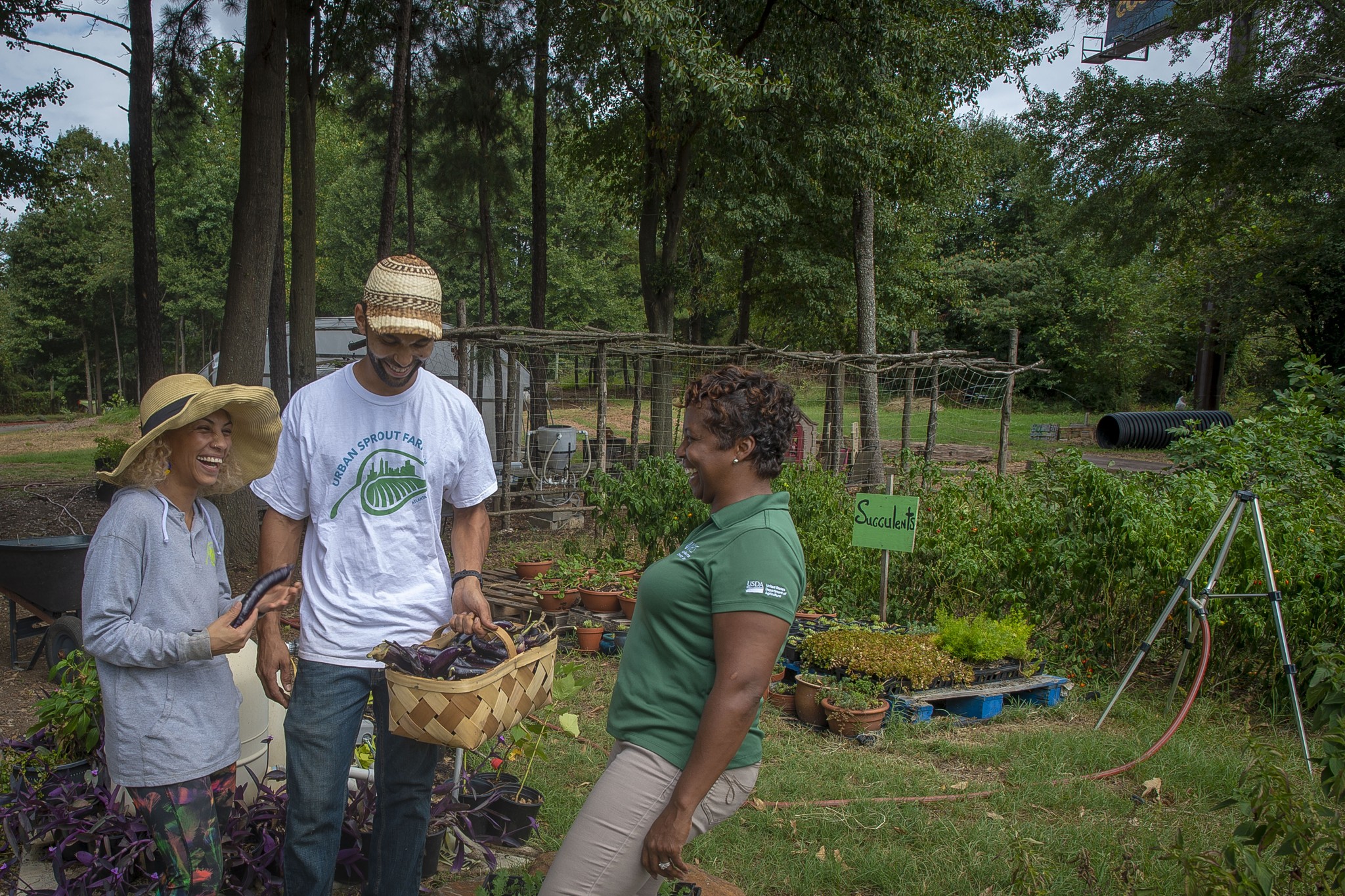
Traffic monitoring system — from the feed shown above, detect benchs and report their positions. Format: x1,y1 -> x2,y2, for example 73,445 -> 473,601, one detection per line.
880,440 -> 993,495
441,462 -> 631,535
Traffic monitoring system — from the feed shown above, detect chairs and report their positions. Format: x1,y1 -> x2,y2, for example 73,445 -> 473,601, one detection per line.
820,443 -> 878,494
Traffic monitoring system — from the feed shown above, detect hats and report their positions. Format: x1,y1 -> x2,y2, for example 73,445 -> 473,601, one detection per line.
362,254 -> 442,340
95,374 -> 283,497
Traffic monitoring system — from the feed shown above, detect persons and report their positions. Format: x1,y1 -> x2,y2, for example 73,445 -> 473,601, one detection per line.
254,252 -> 500,896
79,373 -> 303,896
539,363 -> 807,896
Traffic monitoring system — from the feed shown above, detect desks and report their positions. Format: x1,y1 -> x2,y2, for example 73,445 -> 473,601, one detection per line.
500,468 -> 555,505
568,462 -> 617,475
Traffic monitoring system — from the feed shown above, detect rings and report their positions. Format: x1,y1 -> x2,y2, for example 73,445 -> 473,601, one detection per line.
659,861 -> 671,870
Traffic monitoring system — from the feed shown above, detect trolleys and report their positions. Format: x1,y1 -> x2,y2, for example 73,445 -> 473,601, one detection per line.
0,535 -> 98,685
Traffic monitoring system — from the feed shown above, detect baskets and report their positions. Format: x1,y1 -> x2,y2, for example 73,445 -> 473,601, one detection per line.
385,622 -> 558,751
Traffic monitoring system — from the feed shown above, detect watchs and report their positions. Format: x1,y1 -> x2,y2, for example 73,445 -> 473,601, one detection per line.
450,567 -> 483,589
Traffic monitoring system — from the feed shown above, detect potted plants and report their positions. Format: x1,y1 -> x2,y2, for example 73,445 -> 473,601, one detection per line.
0,540 -> 646,896
761,596 -> 1046,736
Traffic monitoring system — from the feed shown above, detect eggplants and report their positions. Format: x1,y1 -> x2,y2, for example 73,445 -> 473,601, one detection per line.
367,608 -> 559,683
234,566 -> 293,624
